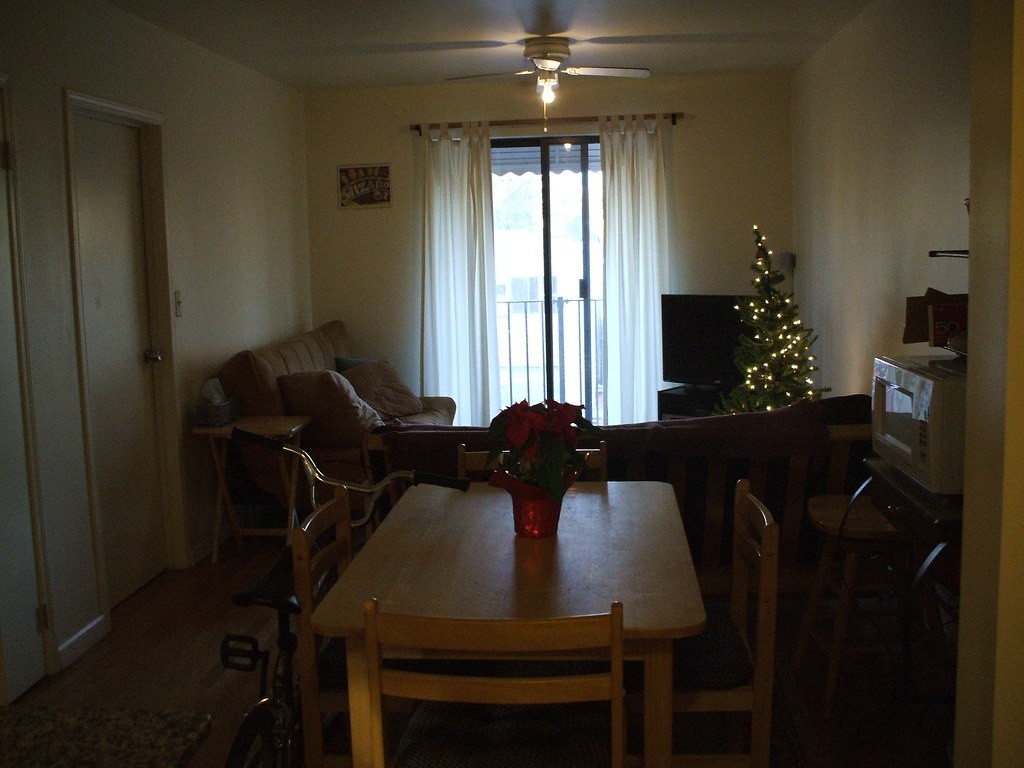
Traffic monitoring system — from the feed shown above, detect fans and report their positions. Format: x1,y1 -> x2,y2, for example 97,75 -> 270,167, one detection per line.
445,36 -> 651,82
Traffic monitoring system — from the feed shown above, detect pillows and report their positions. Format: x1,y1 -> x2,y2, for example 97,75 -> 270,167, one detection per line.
276,369 -> 386,447
340,356 -> 424,421
336,356 -> 375,372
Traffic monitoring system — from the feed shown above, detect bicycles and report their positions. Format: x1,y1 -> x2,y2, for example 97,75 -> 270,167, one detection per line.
196,424 -> 472,768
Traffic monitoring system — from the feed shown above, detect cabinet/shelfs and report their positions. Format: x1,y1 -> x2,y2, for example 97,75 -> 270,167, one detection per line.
866,458 -> 963,768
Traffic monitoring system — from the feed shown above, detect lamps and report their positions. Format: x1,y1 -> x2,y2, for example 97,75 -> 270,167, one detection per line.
537,73 -> 558,104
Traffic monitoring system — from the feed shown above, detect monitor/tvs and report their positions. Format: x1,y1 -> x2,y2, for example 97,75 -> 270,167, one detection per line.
661,293 -> 757,390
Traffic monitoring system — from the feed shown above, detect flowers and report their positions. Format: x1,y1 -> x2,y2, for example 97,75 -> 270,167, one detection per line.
483,399 -> 603,490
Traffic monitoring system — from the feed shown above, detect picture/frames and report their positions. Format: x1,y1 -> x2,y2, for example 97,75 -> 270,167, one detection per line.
337,164 -> 393,209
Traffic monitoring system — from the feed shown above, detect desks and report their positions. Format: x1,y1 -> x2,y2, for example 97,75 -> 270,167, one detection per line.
193,417 -> 310,560
308,483 -> 706,768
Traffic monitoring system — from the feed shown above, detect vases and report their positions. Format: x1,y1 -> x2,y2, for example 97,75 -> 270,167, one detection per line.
490,471 -> 579,536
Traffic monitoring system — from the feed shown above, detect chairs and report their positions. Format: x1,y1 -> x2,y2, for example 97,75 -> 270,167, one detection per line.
627,479 -> 779,767
290,485 -> 429,768
365,599 -> 624,768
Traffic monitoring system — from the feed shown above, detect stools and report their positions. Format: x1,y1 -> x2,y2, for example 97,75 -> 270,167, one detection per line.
791,493 -> 922,717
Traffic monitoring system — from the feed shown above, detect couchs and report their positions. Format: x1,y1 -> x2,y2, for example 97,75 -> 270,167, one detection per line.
365,393 -> 871,578
229,320 -> 456,516
